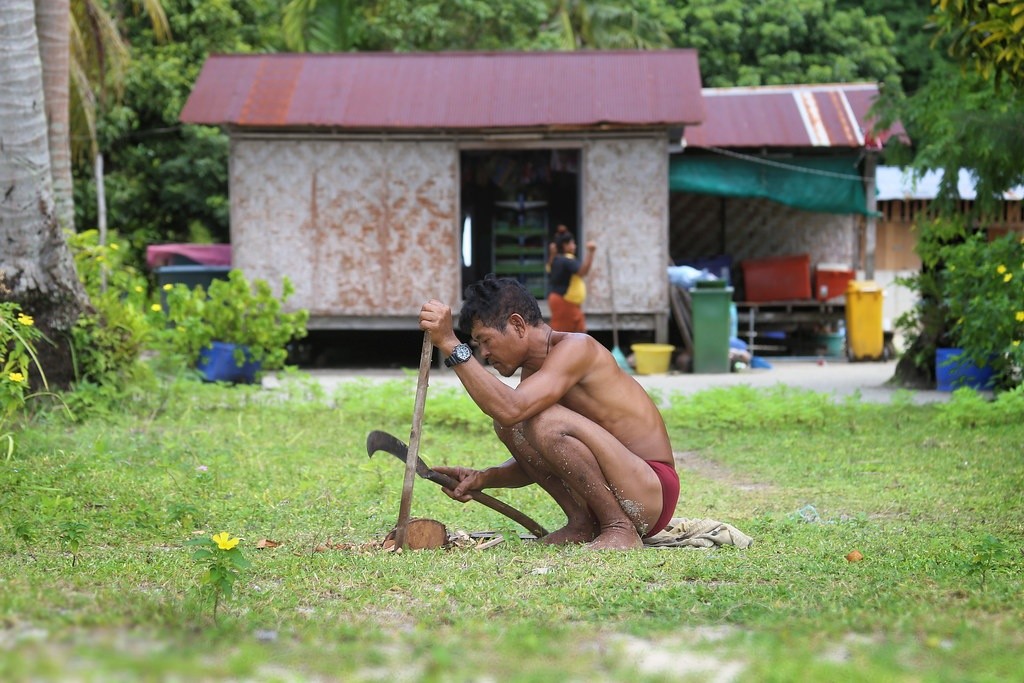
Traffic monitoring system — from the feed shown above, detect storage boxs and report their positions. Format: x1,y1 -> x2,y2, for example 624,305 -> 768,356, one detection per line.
814,263 -> 855,301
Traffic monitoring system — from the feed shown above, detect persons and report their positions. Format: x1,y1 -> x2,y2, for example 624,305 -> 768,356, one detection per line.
546,225 -> 598,333
420,277 -> 680,549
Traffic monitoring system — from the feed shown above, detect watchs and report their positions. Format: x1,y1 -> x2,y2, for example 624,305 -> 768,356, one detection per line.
444,343 -> 472,368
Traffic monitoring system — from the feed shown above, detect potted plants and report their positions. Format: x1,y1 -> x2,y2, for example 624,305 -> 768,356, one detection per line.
197,274 -> 309,384
935,299 -> 999,390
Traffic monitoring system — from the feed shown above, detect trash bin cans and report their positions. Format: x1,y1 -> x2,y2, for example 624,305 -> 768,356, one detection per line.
689,281 -> 734,372
845,279 -> 884,359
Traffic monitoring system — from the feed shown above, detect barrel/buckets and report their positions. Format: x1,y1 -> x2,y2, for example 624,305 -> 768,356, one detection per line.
630,343 -> 675,373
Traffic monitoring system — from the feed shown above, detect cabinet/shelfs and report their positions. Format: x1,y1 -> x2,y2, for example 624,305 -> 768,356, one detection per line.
491,200 -> 550,298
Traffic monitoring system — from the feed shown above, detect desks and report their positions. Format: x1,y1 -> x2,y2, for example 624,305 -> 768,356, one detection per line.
738,300 -> 846,358
